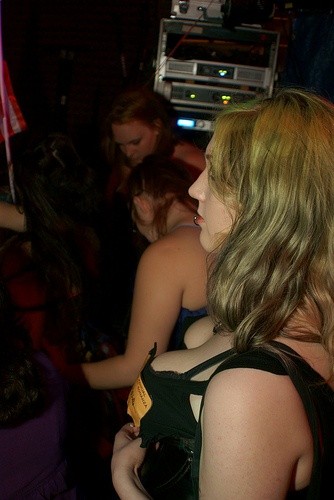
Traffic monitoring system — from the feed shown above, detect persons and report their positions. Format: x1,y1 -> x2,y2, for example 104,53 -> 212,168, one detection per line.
110,89 -> 334,500
81,154 -> 221,390
90,87 -> 206,336
